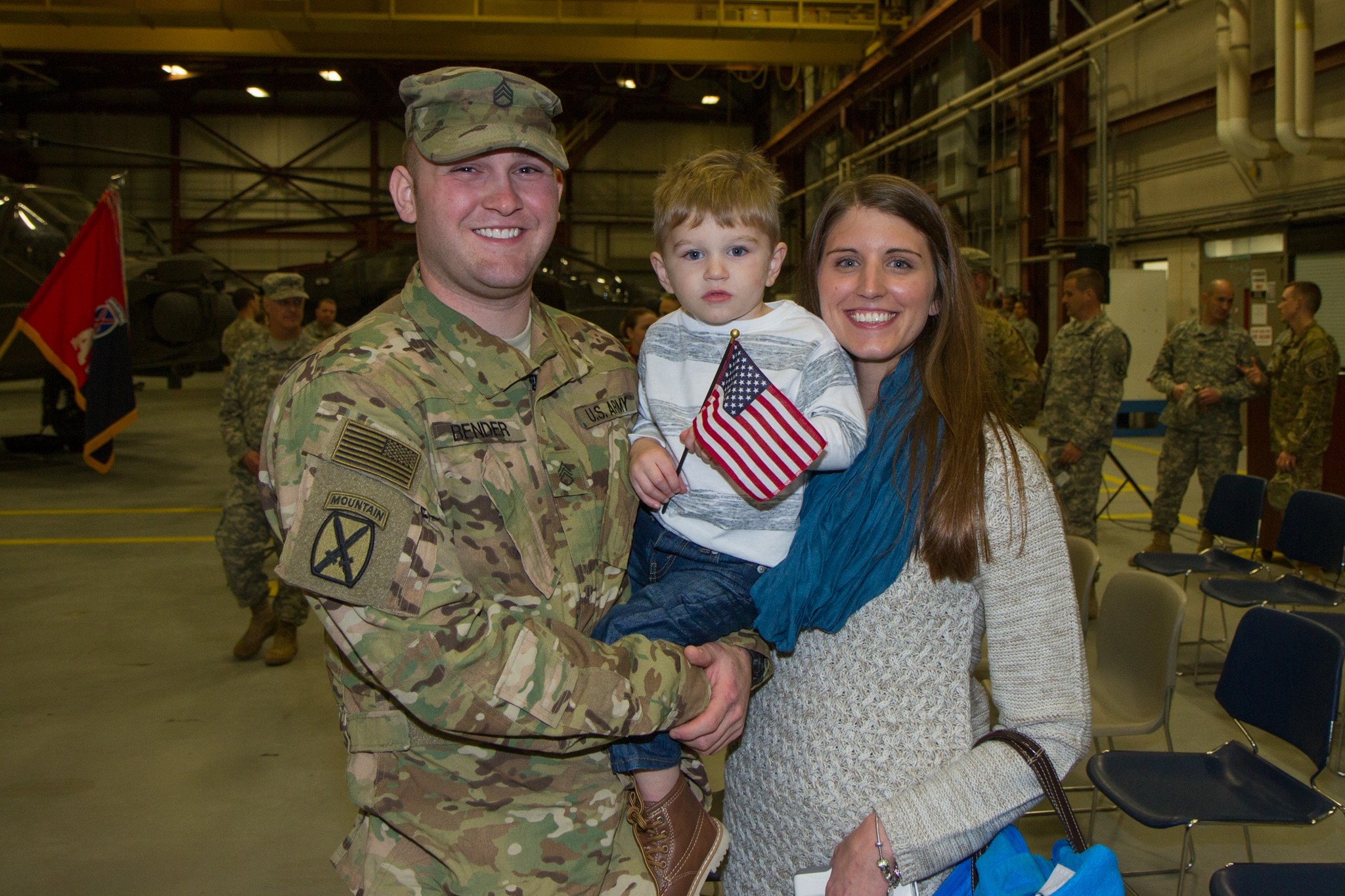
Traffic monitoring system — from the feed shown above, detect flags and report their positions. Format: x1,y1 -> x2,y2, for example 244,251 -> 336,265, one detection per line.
16,188 -> 138,473
693,341 -> 826,502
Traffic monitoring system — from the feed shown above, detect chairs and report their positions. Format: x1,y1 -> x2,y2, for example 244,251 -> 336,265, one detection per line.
974,471 -> 1344,896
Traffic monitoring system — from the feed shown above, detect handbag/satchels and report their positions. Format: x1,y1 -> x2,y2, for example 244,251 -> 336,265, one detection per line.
935,730 -> 1124,896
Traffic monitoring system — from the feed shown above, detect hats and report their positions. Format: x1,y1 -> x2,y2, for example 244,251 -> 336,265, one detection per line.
261,272 -> 308,303
1267,472 -> 1297,511
1173,387 -> 1198,427
399,66 -> 569,170
957,247 -> 1000,278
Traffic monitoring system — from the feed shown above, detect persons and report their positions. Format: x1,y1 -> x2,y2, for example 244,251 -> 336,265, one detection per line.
993,295 -> 1038,352
722,174 -> 1092,896
259,67 -> 776,896
307,298 -> 345,340
957,246 -> 1044,432
1148,279 -> 1264,554
620,293 -> 681,362
221,288 -> 261,360
592,148 -> 870,896
215,273 -> 313,665
1040,268 -> 1132,620
1236,282 -> 1340,584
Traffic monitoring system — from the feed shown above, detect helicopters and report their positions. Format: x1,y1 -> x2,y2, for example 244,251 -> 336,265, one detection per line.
0,127 -> 391,389
161,211 -> 662,338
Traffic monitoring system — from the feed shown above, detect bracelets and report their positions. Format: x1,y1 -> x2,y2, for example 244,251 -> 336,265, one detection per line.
874,813 -> 899,896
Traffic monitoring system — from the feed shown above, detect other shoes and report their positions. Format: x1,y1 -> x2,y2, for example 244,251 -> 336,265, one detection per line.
1088,582 -> 1098,617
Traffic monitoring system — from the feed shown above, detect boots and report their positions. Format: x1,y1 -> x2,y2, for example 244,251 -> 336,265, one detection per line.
234,596 -> 275,660
265,621 -> 297,665
1197,530 -> 1214,552
1128,533 -> 1172,567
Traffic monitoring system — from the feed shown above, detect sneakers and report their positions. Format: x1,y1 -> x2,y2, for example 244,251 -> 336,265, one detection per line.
626,771 -> 728,896
